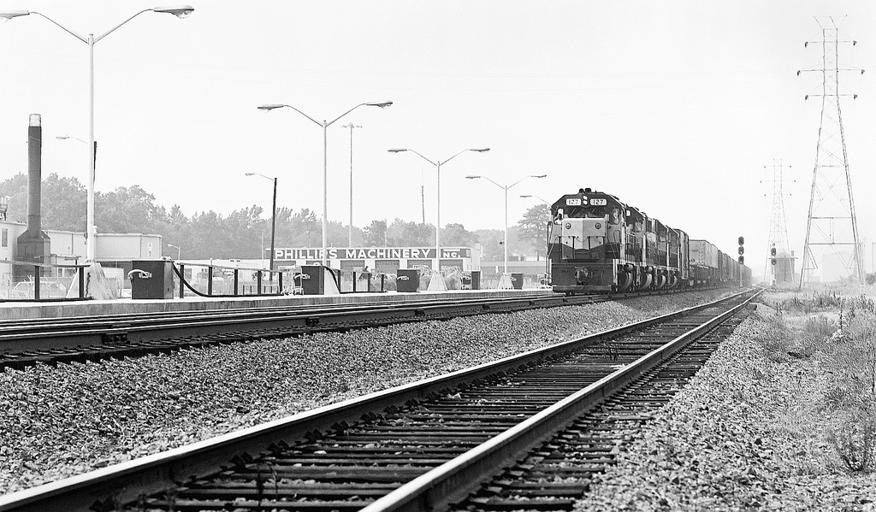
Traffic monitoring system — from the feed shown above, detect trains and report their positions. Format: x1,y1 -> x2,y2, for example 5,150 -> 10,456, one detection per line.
548,188 -> 752,295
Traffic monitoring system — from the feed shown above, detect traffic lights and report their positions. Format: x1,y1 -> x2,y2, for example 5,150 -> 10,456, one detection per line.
738,237 -> 745,265
771,248 -> 777,266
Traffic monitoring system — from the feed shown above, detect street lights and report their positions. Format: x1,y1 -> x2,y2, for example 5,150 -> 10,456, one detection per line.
388,148 -> 491,271
243,171 -> 277,291
1,6 -> 193,261
258,102 -> 392,266
468,176 -> 545,272
